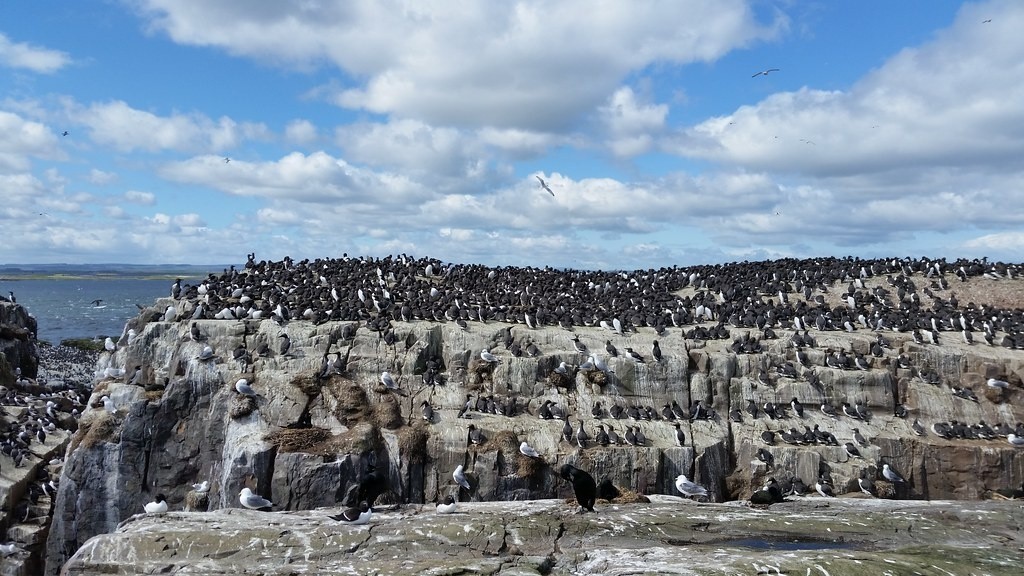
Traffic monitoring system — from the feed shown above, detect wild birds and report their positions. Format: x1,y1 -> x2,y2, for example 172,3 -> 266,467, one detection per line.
93,249 -> 1024,517
0,365 -> 88,553
62,130 -> 69,136
751,67 -> 780,78
5,290 -> 17,303
326,501 -> 370,525
982,18 -> 992,23
536,175 -> 555,197
